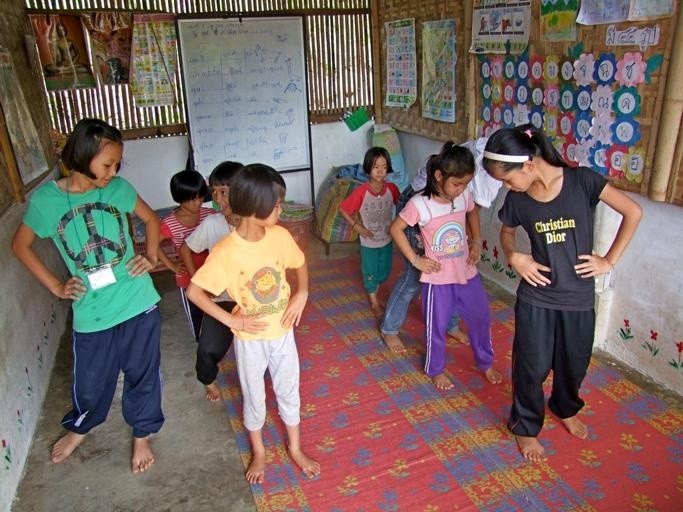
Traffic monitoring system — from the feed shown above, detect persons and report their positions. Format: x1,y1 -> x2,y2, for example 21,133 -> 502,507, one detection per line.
158,169 -> 219,344
481,123 -> 643,462
390,140 -> 503,390
185,164 -> 322,485
379,137 -> 505,352
179,161 -> 245,402
11,118 -> 165,475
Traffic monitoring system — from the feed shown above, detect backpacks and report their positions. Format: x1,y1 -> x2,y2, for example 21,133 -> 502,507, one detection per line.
337,146 -> 401,318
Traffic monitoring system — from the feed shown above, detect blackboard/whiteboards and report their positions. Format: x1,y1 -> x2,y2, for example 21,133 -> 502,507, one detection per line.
174,14 -> 313,187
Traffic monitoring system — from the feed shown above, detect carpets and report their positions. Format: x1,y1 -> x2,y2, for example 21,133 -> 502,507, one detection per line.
215,245 -> 683,512
